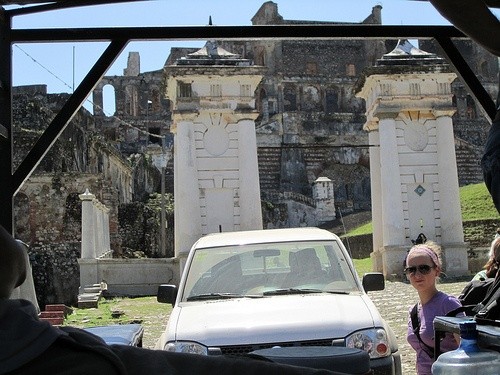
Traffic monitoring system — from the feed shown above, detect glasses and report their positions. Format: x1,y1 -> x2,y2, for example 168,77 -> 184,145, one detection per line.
404,265 -> 434,276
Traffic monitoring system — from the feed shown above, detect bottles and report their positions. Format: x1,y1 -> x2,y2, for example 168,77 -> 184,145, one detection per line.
431,321 -> 500,375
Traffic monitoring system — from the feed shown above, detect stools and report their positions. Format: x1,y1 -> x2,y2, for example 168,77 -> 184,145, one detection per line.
81,324 -> 144,347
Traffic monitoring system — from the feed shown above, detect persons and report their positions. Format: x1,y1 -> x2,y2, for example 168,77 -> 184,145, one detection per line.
0,224 -> 127,375
458,234 -> 500,354
406,240 -> 468,375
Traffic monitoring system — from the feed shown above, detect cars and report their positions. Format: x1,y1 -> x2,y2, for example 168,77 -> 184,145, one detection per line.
153,227 -> 402,375
